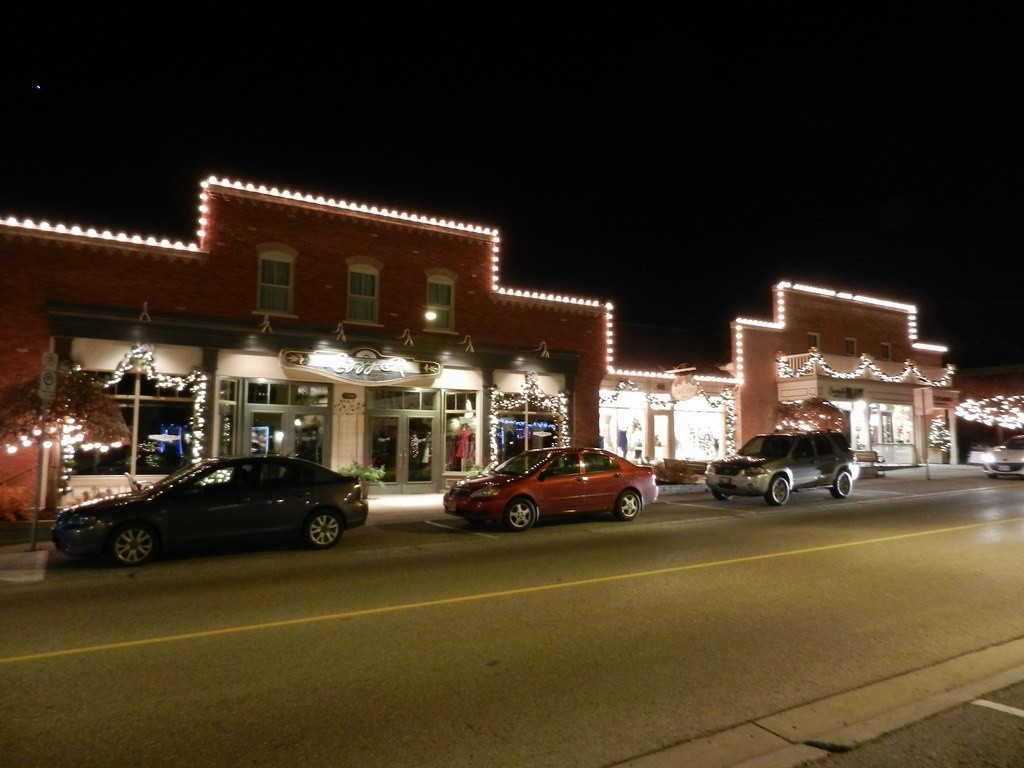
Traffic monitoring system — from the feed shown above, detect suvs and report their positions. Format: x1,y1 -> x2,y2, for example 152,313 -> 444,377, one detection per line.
704,427 -> 859,507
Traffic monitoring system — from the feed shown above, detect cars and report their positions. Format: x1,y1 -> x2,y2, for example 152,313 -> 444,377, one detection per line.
444,447 -> 659,532
51,455 -> 369,567
966,443 -> 989,465
982,435 -> 1024,480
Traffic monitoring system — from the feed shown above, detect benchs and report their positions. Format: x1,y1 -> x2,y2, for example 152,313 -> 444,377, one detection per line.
855,450 -> 886,476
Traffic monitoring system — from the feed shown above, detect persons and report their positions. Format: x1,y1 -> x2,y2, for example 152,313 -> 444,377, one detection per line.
455,423 -> 474,458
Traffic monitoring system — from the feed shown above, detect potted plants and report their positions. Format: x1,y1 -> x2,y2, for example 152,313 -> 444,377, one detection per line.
927,419 -> 952,464
340,461 -> 385,499
